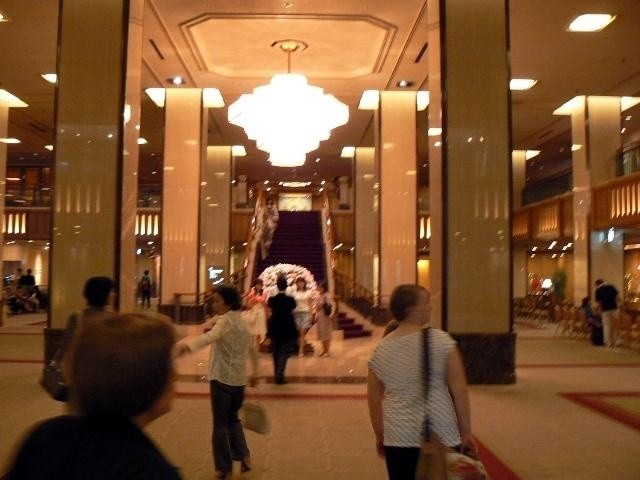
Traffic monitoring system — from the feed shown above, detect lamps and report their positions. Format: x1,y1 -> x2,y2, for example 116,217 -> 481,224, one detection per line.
226,38 -> 351,170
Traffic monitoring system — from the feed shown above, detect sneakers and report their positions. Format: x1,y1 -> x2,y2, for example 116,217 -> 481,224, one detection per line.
214,468 -> 234,479
240,456 -> 251,472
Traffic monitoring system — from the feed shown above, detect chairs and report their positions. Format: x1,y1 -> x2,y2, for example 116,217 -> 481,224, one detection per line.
553,305 -> 584,341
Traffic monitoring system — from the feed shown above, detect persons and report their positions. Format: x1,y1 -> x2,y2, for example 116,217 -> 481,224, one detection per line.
592,279 -> 624,350
311,281 -> 337,359
50,277 -> 117,416
0,311 -> 185,480
140,270 -> 152,310
267,277 -> 300,383
173,281 -> 265,480
241,279 -> 269,351
567,305 -> 576,326
3,267 -> 48,314
366,286 -> 480,480
290,277 -> 314,357
257,196 -> 281,252
582,295 -> 605,346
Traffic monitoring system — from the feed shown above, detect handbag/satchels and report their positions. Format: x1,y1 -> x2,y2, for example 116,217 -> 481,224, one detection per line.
40,358 -> 68,402
242,402 -> 269,434
415,428 -> 489,479
324,303 -> 332,316
